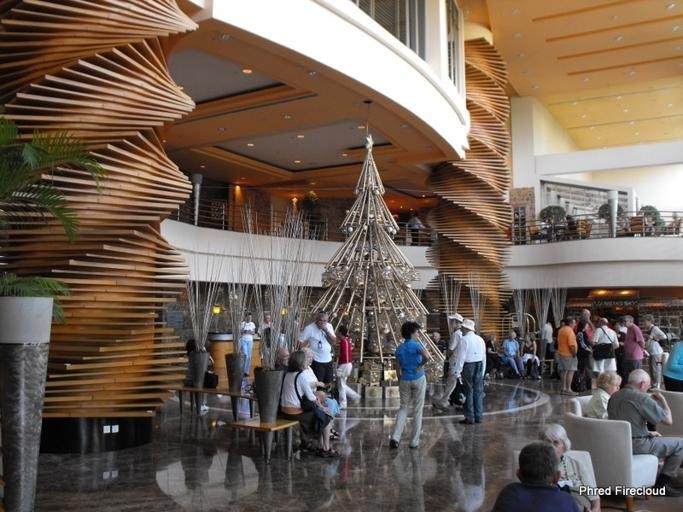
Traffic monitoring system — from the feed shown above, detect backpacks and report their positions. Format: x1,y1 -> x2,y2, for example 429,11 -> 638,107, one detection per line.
649,326 -> 670,347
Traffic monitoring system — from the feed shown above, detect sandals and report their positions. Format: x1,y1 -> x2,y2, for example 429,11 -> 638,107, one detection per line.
560,389 -> 580,396
301,429 -> 340,457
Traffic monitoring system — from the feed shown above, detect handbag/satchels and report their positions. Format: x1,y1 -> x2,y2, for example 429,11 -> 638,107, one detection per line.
448,376 -> 468,406
334,364 -> 351,380
527,359 -> 539,377
592,343 -> 615,361
571,370 -> 586,392
300,393 -> 318,410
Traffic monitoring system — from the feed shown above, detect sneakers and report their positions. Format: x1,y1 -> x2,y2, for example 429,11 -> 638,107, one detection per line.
652,474 -> 683,499
650,382 -> 661,392
460,417 -> 483,425
390,439 -> 419,449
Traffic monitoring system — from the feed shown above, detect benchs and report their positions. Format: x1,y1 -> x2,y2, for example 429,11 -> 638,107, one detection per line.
179,387 -> 258,421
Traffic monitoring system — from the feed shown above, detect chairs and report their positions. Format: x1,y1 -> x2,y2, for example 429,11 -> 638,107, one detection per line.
571,224 -> 592,240
528,226 -> 551,244
512,390 -> 682,512
631,217 -> 643,237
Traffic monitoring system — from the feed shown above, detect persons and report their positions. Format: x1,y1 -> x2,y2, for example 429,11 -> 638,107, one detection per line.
432,307 -> 683,512
540,216 -> 553,242
561,214 -> 577,240
390,321 -> 431,449
187,335 -> 215,411
237,310 -> 364,458
407,211 -> 426,245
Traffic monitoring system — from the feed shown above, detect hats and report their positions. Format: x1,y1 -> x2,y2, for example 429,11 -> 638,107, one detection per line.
459,319 -> 478,332
448,313 -> 464,323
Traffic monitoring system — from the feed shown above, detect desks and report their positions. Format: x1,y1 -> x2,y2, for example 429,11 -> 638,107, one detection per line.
233,416 -> 300,464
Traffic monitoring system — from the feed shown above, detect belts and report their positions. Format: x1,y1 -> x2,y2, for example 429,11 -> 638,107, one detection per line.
632,436 -> 650,441
